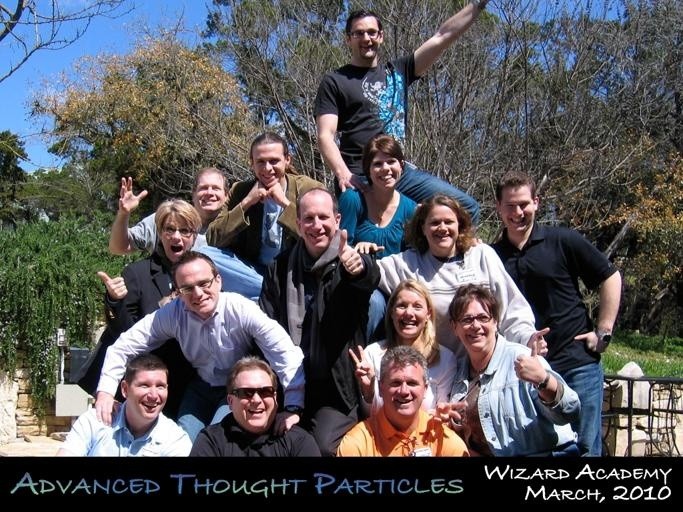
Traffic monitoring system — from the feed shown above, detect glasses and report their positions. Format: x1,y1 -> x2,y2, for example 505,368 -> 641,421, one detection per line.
347,30 -> 381,38
455,312 -> 492,324
178,276 -> 216,295
162,223 -> 193,237
229,386 -> 275,399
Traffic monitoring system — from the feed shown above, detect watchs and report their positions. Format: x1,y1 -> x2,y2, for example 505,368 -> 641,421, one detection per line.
595,328 -> 612,347
286,403 -> 304,418
533,372 -> 551,392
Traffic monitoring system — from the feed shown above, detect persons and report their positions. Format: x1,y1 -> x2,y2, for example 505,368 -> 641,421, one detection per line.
197,132 -> 327,303
312,0 -> 494,229
488,172 -> 621,457
348,280 -> 456,428
249,186 -> 381,457
449,284 -> 581,457
96,251 -> 307,447
110,167 -> 228,257
352,193 -> 550,358
333,134 -> 419,346
189,357 -> 321,457
58,353 -> 192,457
96,198 -> 201,421
334,345 -> 470,457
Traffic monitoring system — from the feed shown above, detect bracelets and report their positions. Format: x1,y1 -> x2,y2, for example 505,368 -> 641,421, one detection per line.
470,0 -> 486,10
